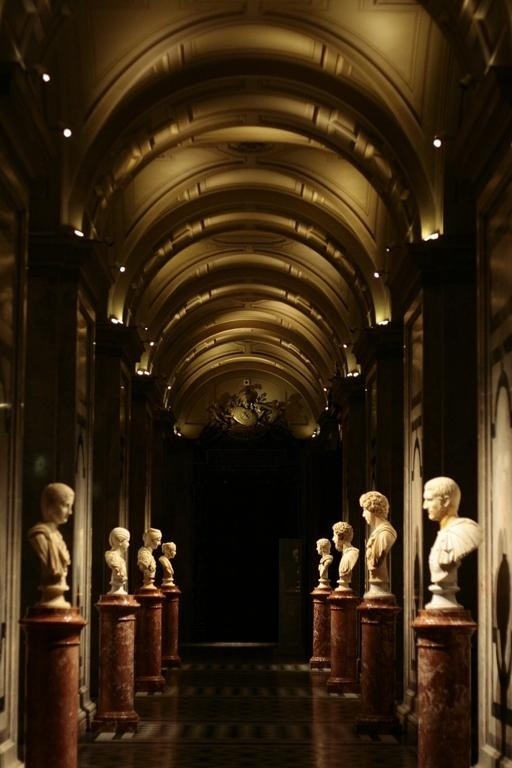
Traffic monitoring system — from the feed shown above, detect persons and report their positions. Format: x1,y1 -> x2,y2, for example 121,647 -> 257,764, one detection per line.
422,476 -> 478,609
332,522 -> 360,592
137,528 -> 161,590
27,482 -> 75,608
105,528 -> 131,596
313,537 -> 333,588
360,490 -> 398,594
158,542 -> 178,587
